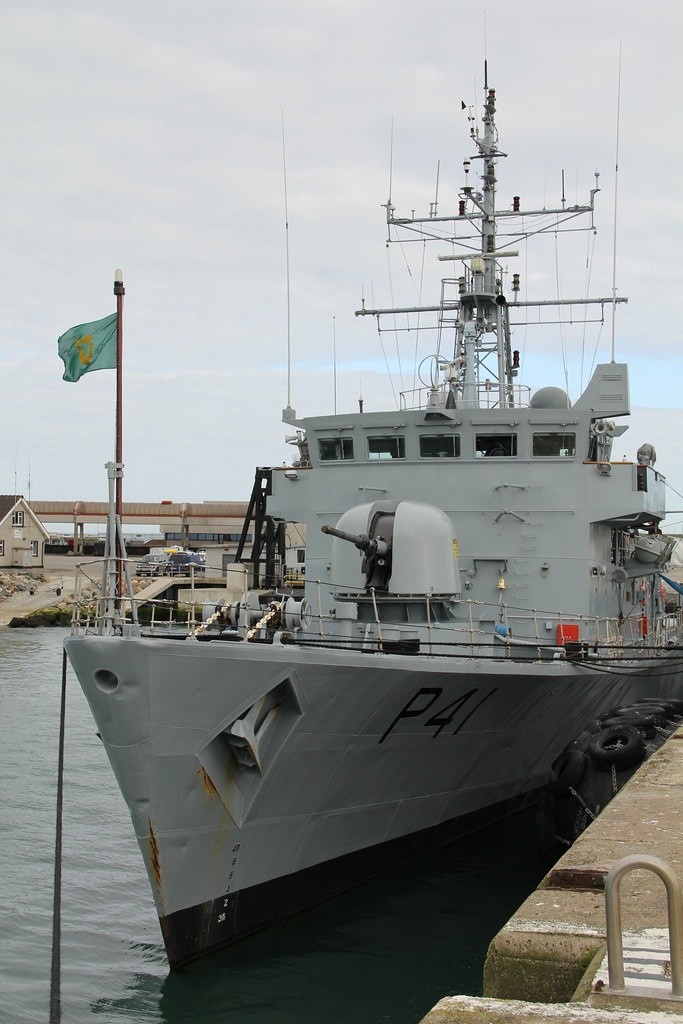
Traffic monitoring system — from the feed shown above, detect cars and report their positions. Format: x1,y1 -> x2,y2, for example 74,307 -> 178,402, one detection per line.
161,553 -> 201,577
134,554 -> 167,576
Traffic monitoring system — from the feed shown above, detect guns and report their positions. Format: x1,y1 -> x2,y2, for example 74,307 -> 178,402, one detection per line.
320,525 -> 389,588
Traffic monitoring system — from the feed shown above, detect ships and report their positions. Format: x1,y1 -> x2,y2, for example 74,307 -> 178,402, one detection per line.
59,5 -> 683,971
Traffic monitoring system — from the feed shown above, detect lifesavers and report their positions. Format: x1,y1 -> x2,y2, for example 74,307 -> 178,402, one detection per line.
660,586 -> 664,601
642,616 -> 647,636
642,578 -> 647,605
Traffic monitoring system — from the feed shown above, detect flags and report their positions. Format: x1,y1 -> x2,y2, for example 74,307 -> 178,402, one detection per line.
57,308 -> 117,382
